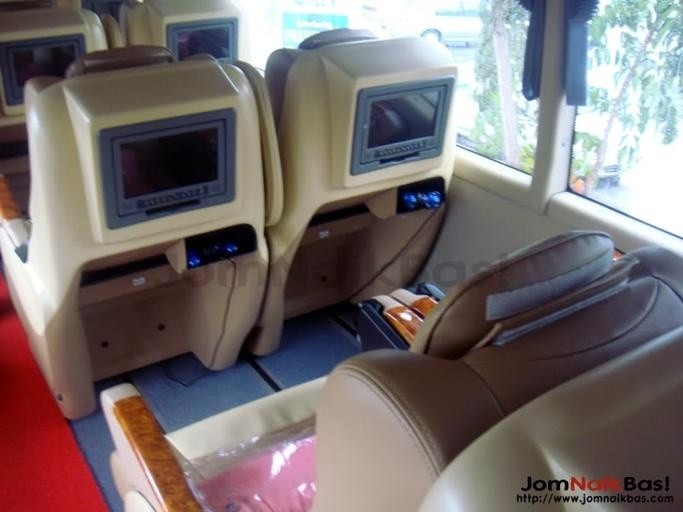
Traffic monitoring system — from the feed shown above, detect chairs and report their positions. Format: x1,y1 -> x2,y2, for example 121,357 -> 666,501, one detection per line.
0,2 -> 681,511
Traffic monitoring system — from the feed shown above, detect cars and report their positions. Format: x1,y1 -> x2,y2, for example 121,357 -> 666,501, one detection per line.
362,0 -> 486,48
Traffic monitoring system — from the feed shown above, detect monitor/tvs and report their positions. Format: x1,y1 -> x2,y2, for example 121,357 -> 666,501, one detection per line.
96,106 -> 237,229
350,76 -> 455,175
168,19 -> 239,66
0,33 -> 86,107
